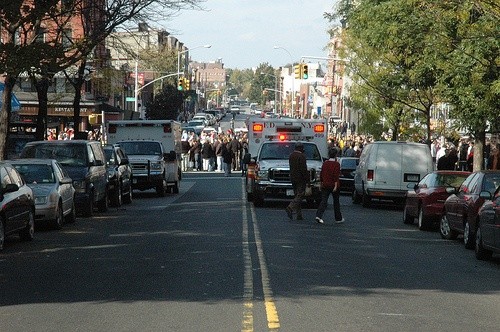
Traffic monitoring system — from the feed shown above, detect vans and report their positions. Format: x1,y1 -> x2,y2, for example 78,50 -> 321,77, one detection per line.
20,139 -> 109,218
351,141 -> 433,207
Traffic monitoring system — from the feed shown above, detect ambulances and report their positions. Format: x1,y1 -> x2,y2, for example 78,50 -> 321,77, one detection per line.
107,120 -> 182,197
243,116 -> 328,208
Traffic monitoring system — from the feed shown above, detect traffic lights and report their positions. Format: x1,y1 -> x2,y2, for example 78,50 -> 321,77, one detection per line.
178,78 -> 189,91
294,64 -> 308,79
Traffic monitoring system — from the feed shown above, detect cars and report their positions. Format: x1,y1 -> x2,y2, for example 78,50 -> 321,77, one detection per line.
181,120 -> 217,135
337,157 -> 360,191
192,107 -> 226,126
230,106 -> 239,113
403,171 -> 474,230
474,184 -> 500,260
0,161 -> 36,251
250,103 -> 292,119
437,170 -> 500,249
2,159 -> 76,226
329,116 -> 341,122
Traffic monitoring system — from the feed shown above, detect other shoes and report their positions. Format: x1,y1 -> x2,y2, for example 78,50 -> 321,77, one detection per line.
296,215 -> 304,220
336,217 -> 344,224
285,207 -> 293,219
315,217 -> 324,224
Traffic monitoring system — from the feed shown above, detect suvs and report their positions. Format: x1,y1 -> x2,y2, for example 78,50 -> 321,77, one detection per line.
100,144 -> 133,206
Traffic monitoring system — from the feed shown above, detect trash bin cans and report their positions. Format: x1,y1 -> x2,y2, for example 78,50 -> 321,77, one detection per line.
458,161 -> 467,171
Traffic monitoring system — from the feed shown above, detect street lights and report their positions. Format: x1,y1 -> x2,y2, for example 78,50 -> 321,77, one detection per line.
261,46 -> 294,116
135,44 -> 212,113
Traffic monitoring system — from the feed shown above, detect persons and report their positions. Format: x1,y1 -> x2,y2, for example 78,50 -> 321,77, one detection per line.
431,135 -> 500,172
286,142 -> 308,220
180,112 -> 249,177
316,148 -> 346,224
26,126 -> 101,142
314,113 -> 374,157
